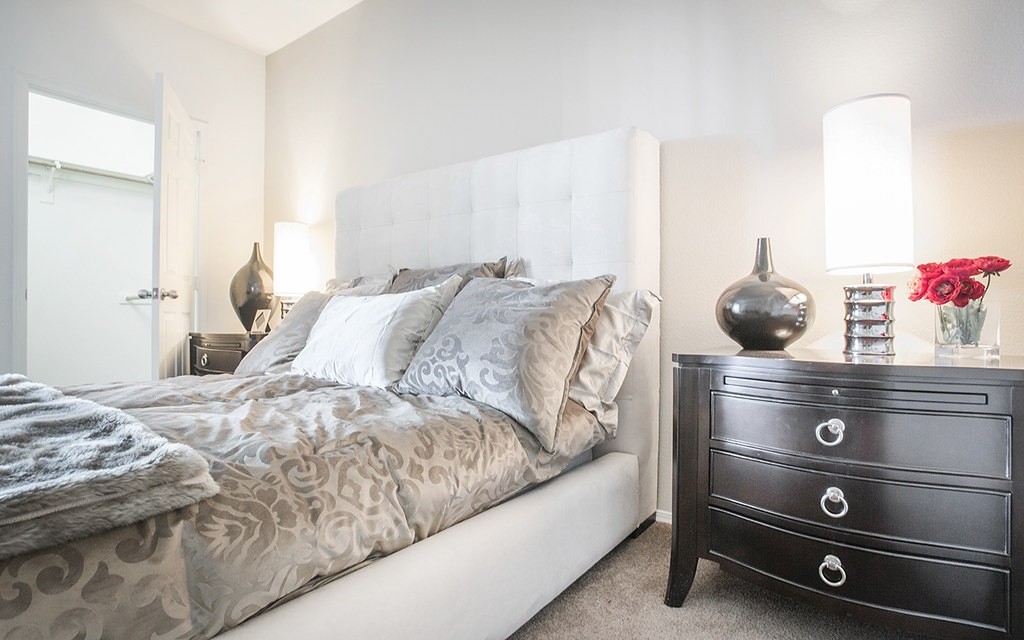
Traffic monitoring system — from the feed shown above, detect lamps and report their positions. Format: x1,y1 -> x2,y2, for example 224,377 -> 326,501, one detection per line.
272,220 -> 312,318
822,92 -> 918,363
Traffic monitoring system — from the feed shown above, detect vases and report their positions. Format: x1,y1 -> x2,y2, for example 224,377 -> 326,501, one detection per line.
716,237 -> 815,350
230,242 -> 280,332
935,298 -> 1002,366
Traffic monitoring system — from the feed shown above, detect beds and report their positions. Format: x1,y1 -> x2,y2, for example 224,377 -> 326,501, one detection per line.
0,128 -> 662,640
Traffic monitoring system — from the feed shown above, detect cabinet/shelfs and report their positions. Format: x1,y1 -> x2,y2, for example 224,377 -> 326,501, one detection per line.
664,351 -> 1023,640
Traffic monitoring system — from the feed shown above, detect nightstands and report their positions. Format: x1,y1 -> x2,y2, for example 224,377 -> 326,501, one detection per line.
188,332 -> 267,376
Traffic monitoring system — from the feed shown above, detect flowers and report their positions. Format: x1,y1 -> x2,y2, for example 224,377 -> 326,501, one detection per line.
905,256 -> 1013,342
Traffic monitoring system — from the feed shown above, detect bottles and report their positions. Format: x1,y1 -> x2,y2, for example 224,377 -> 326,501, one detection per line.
715,237 -> 816,351
230,242 -> 280,334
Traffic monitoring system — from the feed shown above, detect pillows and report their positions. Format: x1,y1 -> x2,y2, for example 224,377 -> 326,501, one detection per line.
389,257 -> 508,297
286,273 -> 464,389
325,278 -> 387,292
568,288 -> 663,435
391,274 -> 616,455
234,283 -> 393,378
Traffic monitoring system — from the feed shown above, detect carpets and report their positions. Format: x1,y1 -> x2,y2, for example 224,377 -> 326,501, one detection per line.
506,522 -> 930,640
